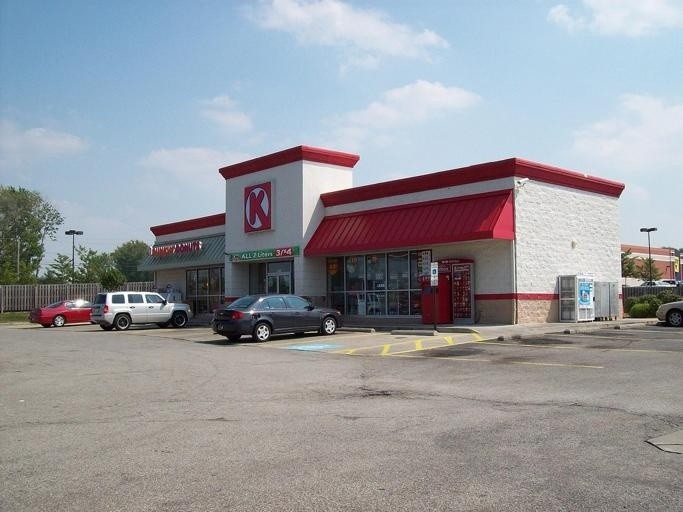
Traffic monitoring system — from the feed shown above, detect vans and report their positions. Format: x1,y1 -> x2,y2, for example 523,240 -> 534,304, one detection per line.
90,290 -> 195,332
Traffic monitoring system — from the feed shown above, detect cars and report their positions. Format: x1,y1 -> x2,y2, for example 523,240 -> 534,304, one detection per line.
655,300 -> 682,327
29,298 -> 99,328
209,293 -> 344,344
640,278 -> 682,287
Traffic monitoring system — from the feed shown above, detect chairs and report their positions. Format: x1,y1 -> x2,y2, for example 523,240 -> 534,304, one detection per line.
355,292 -> 387,315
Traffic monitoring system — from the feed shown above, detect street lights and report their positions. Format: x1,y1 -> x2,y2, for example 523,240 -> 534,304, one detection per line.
639,228 -> 657,286
65,229 -> 84,284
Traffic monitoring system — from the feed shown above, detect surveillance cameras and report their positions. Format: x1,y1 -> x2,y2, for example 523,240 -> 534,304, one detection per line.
517,178 -> 529,185
